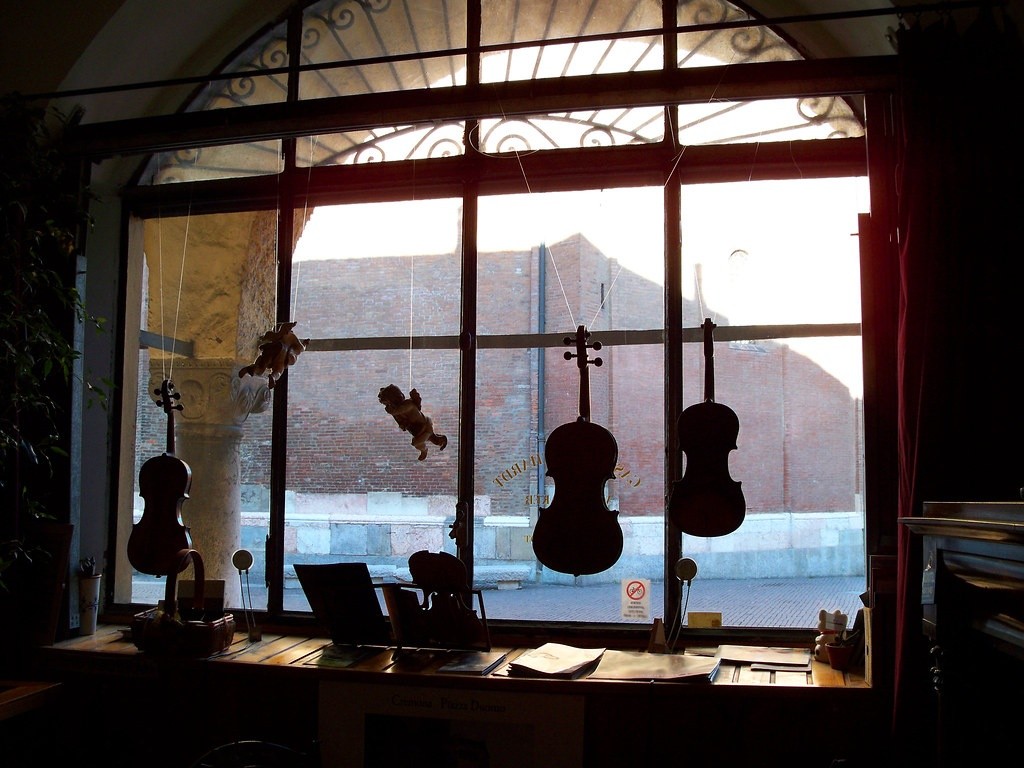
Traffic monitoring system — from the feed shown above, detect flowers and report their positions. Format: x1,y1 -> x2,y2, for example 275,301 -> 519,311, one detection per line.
830,614 -> 848,646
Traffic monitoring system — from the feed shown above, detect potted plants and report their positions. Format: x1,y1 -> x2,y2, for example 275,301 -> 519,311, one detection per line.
827,643 -> 847,670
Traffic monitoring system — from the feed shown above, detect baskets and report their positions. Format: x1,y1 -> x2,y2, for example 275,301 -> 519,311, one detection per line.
132,547 -> 235,657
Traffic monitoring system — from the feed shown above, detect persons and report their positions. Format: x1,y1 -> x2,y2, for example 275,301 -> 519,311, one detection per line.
238,322 -> 310,389
378,385 -> 448,459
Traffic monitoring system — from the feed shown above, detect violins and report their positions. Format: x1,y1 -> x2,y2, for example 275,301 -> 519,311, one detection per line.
126,378 -> 195,578
532,325 -> 626,579
670,318 -> 748,540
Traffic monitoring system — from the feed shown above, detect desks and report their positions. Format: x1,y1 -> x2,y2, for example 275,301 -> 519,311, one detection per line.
42,624 -> 866,768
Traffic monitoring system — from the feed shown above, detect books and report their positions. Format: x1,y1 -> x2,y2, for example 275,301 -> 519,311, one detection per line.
437,644 -> 607,678
